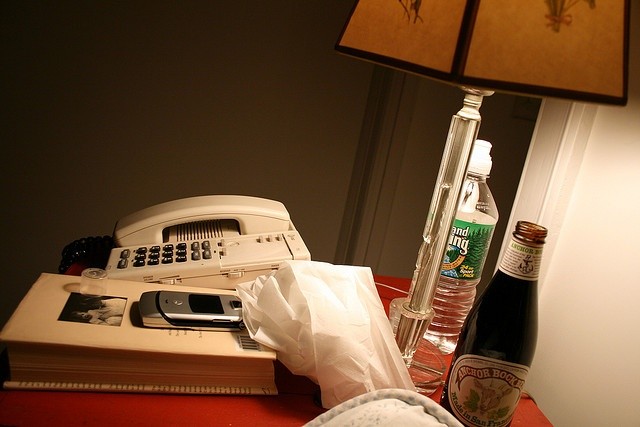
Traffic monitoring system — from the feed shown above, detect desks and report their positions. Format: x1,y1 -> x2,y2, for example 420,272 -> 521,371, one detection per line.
1,256 -> 557,427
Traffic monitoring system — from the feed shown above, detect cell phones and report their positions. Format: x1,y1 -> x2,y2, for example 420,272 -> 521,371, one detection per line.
138,291 -> 246,333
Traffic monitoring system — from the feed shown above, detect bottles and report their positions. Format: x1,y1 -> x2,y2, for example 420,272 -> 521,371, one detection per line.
419,138 -> 500,354
440,220 -> 549,427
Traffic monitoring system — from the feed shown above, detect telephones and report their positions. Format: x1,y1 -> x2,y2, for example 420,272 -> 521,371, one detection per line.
58,195 -> 312,290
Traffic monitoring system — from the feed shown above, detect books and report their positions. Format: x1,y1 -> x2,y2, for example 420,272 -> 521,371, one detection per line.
0,273 -> 281,397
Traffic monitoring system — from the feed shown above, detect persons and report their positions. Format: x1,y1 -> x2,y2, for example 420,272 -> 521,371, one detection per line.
77,295 -> 127,312
70,309 -> 123,326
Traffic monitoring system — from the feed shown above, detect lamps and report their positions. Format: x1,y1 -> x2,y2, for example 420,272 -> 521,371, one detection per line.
338,0 -> 631,386
